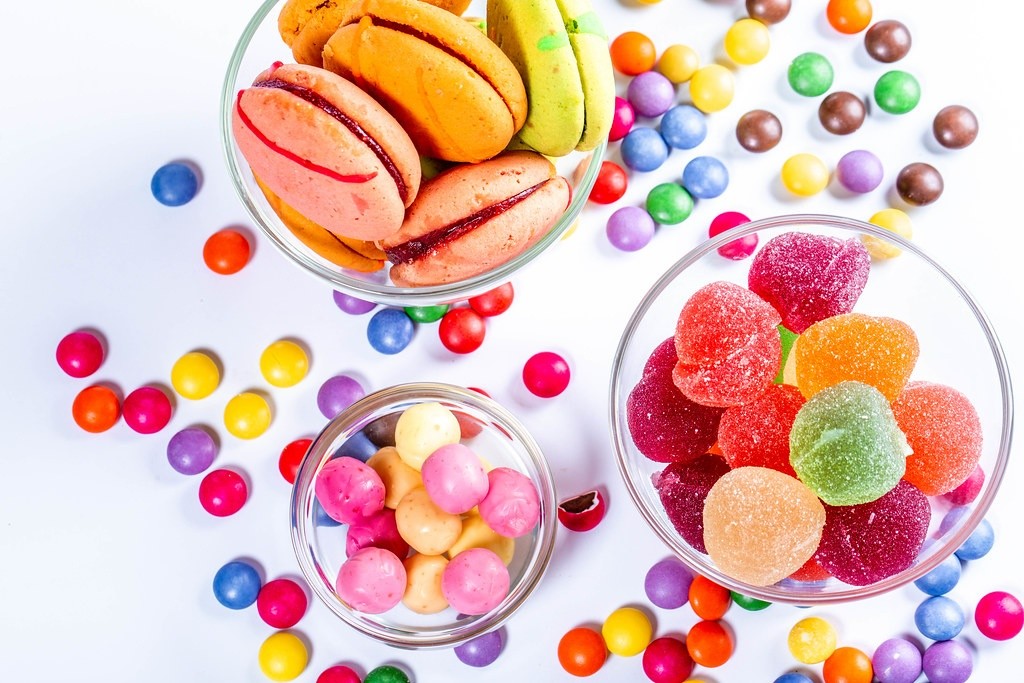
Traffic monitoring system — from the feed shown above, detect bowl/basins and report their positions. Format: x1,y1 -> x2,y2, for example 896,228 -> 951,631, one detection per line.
609,214 -> 1013,608
289,381 -> 558,650
220,0 -> 609,306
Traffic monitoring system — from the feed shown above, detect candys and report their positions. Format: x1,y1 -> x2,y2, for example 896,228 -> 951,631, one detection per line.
608,0 -> 1023,683
56,168 -> 608,683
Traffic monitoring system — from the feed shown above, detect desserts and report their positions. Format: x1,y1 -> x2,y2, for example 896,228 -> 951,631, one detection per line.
229,0 -> 608,289
